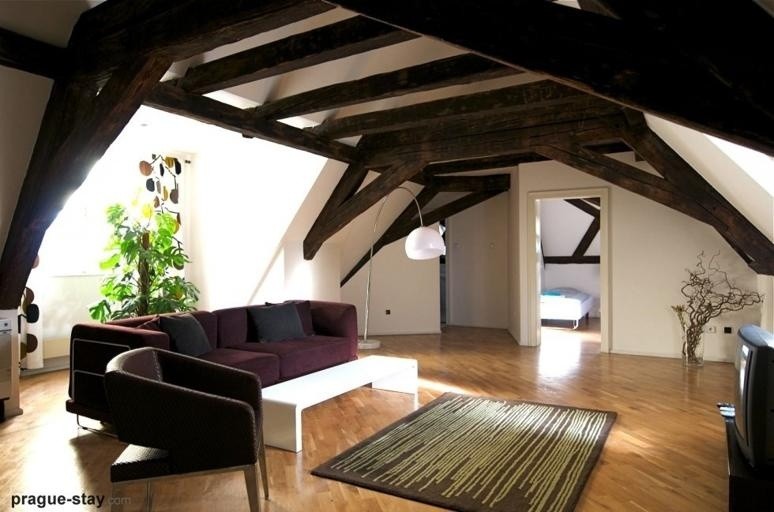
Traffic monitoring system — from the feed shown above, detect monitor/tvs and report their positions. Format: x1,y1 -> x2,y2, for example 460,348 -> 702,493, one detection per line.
733,324 -> 774,469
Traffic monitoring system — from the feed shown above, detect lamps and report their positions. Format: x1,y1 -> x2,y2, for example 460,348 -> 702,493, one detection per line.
358,187 -> 445,350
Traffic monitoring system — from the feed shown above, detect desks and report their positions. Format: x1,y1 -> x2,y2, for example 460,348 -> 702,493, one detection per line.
717,403 -> 774,512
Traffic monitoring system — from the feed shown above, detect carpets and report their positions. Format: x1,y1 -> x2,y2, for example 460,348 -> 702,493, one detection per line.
310,392 -> 618,512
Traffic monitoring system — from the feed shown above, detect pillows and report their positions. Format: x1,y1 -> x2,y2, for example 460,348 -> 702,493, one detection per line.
247,302 -> 306,343
266,302 -> 315,337
157,313 -> 211,357
136,317 -> 161,332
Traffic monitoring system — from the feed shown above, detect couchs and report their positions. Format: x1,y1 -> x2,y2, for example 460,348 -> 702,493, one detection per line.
67,300 -> 357,439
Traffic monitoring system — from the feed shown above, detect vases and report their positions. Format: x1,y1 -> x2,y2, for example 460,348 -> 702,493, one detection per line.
682,329 -> 704,368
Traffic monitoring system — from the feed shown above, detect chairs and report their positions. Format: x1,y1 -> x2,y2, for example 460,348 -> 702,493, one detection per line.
104,347 -> 269,512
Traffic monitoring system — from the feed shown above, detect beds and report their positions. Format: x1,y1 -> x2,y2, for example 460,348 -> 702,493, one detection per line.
541,288 -> 592,330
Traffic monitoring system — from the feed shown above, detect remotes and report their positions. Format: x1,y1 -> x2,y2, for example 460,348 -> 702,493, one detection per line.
717,402 -> 736,408
720,406 -> 735,412
720,411 -> 735,418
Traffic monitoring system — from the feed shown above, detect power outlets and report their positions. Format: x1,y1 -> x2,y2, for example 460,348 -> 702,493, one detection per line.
708,326 -> 715,333
